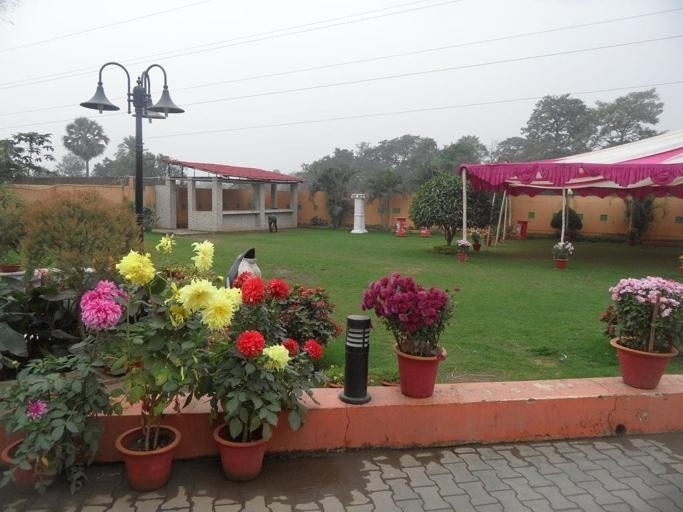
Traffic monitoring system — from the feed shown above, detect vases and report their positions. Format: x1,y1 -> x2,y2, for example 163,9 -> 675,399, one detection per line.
458,252 -> 467,261
393,340 -> 447,398
609,336 -> 679,389
553,258 -> 568,269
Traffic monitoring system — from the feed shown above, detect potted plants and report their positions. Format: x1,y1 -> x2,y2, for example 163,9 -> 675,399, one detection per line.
624,224 -> 638,247
471,230 -> 482,251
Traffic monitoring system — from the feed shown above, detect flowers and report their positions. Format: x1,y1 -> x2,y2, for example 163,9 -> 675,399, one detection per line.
362,271 -> 455,356
0,230 -> 344,496
455,240 -> 471,255
551,239 -> 575,259
600,275 -> 683,352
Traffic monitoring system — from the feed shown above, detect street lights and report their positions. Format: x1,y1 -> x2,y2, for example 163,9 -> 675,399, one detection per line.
77,60 -> 185,253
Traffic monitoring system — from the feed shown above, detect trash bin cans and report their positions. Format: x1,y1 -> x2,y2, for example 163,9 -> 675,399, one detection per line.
225,247 -> 261,289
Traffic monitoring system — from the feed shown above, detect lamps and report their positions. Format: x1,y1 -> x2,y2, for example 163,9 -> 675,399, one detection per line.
339,314 -> 372,406
